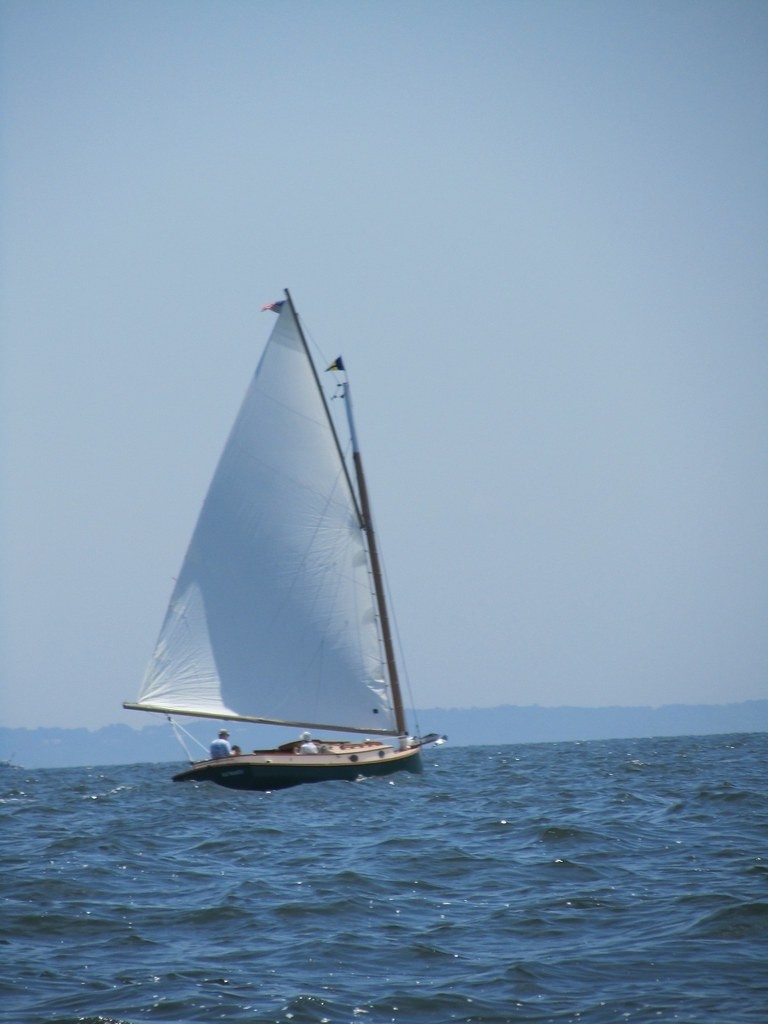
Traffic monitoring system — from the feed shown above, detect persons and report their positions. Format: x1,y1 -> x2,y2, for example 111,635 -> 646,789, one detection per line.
299,732 -> 318,754
209,729 -> 236,760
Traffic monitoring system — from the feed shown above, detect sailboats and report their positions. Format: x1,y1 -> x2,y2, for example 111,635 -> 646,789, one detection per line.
124,287 -> 449,790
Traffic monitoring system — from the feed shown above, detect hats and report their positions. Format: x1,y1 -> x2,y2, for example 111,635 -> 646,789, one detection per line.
217,729 -> 230,736
300,732 -> 312,741
